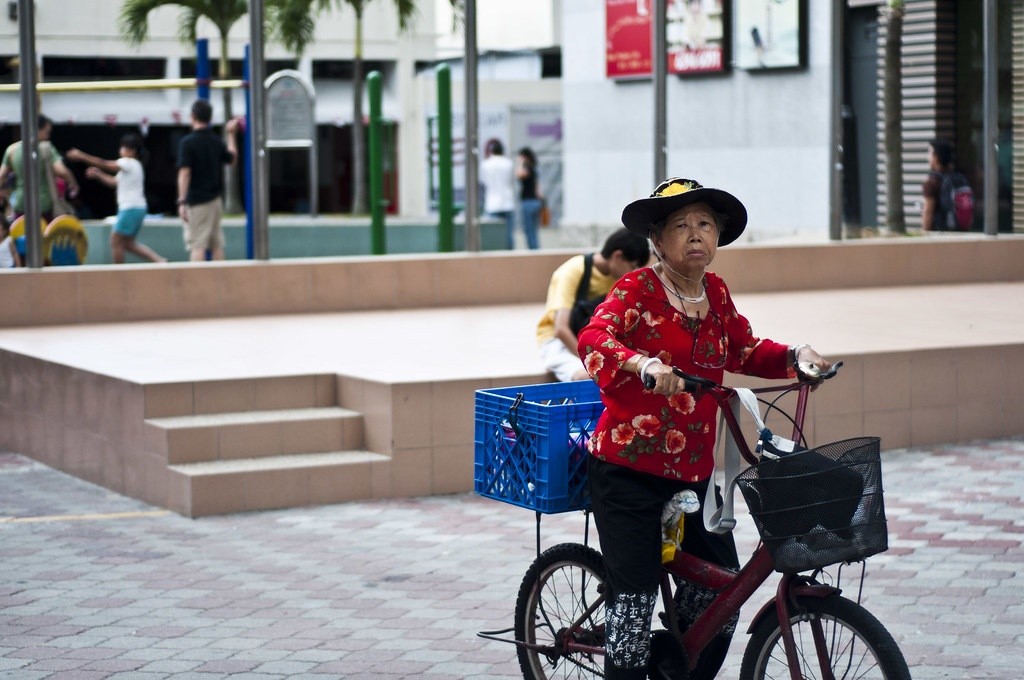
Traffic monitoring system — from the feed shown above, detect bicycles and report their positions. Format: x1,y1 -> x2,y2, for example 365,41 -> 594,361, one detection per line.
475,362 -> 912,680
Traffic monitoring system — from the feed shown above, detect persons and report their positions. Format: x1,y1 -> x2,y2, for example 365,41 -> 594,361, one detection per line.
516,147 -> 541,249
577,177 -> 831,680
177,100 -> 240,261
921,141 -> 966,231
481,139 -> 516,249
0,216 -> 22,268
537,227 -> 650,382
0,114 -> 80,221
66,134 -> 169,264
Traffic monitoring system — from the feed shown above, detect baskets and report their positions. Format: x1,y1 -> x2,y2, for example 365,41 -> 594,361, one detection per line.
736,436 -> 889,573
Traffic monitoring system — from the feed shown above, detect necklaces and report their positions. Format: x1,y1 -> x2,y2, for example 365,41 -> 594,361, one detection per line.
652,265 -> 706,303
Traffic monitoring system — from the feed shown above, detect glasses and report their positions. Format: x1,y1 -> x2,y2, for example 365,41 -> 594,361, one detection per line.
691,310 -> 727,369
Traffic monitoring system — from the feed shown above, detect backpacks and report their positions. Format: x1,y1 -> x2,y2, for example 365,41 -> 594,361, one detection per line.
930,171 -> 976,232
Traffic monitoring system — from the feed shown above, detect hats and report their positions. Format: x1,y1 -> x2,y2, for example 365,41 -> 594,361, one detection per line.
622,177 -> 749,283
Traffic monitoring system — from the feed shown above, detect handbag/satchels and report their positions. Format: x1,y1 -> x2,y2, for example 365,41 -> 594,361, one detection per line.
746,434 -> 865,553
51,199 -> 75,223
568,252 -> 607,336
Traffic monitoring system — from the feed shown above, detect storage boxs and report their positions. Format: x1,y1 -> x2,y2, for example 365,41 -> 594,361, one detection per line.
474,379 -> 605,515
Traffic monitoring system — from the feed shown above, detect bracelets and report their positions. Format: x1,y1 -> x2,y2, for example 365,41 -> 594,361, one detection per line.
790,344 -> 806,361
177,199 -> 186,204
634,354 -> 662,383
